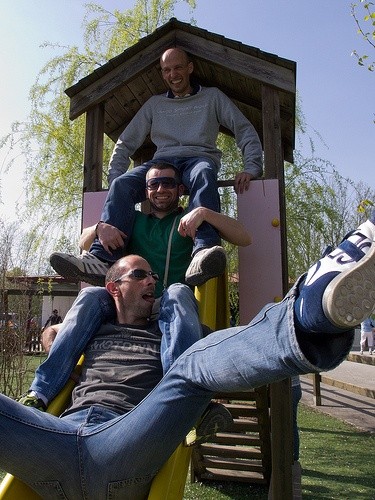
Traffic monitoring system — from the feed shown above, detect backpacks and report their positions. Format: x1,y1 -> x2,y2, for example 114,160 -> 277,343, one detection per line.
148,296 -> 162,322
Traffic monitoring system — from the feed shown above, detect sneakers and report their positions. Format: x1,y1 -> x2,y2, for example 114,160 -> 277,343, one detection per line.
183,401 -> 233,448
185,245 -> 229,287
14,393 -> 47,412
295,210 -> 375,334
49,249 -> 109,287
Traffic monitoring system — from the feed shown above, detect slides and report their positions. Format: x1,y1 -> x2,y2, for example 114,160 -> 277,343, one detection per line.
0,278 -> 216,500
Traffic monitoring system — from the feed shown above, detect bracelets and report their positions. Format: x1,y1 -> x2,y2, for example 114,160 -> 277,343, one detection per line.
95,221 -> 105,237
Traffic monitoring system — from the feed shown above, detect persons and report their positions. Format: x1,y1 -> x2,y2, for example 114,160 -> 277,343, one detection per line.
359,318 -> 374,355
1,212 -> 375,500
50,47 -> 264,286
10,162 -> 252,447
24,309 -> 62,353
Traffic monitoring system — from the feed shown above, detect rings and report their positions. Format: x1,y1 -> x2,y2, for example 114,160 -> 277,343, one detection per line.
182,227 -> 187,231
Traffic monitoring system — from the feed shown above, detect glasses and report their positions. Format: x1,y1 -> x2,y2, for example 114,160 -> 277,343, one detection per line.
114,269 -> 160,283
146,177 -> 177,190
